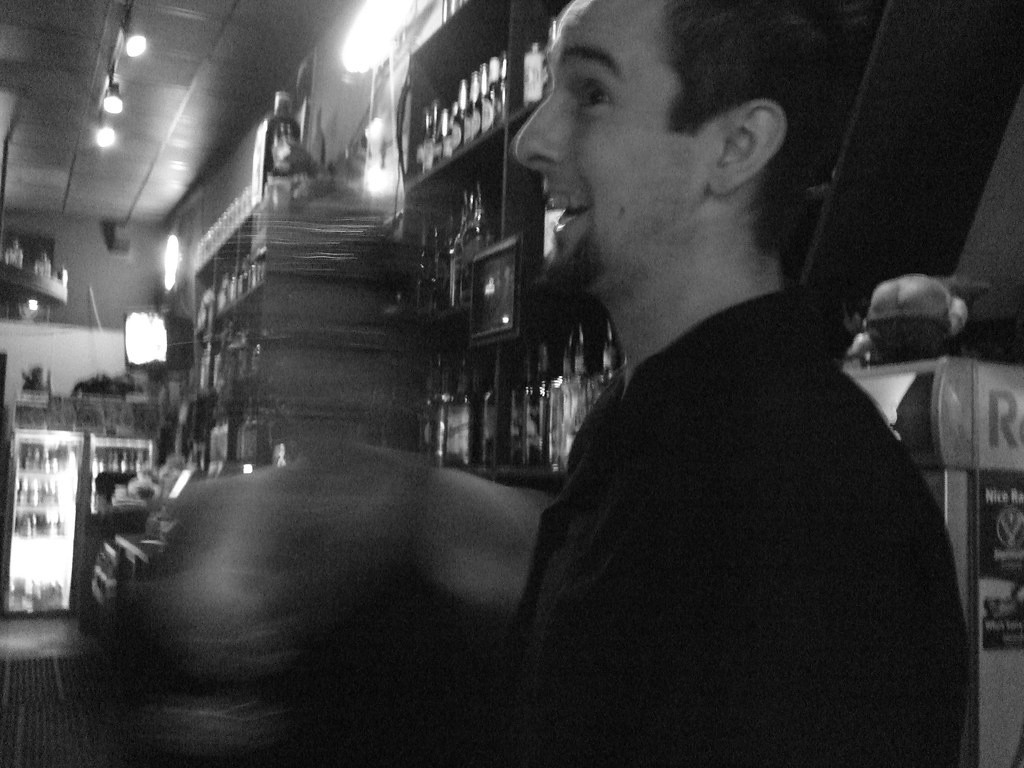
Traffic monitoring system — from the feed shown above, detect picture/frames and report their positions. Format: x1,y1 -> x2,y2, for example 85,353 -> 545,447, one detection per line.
468,230 -> 526,350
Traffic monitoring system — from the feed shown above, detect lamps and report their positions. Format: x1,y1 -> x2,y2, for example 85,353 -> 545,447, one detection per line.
94,11 -> 145,147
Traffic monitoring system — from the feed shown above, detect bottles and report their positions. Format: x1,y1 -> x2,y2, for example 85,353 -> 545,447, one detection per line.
418,186 -> 495,306
19,440 -> 147,537
252,91 -> 299,205
34,254 -> 51,276
418,43 -> 544,173
5,241 -> 23,269
432,317 -> 618,471
228,264 -> 265,301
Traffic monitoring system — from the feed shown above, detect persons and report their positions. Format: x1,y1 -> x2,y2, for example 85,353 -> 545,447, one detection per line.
34,249 -> 51,277
168,0 -> 981,768
4,236 -> 23,269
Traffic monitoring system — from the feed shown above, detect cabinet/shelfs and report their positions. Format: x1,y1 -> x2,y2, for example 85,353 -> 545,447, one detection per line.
400,0 -> 627,498
191,210 -> 402,470
